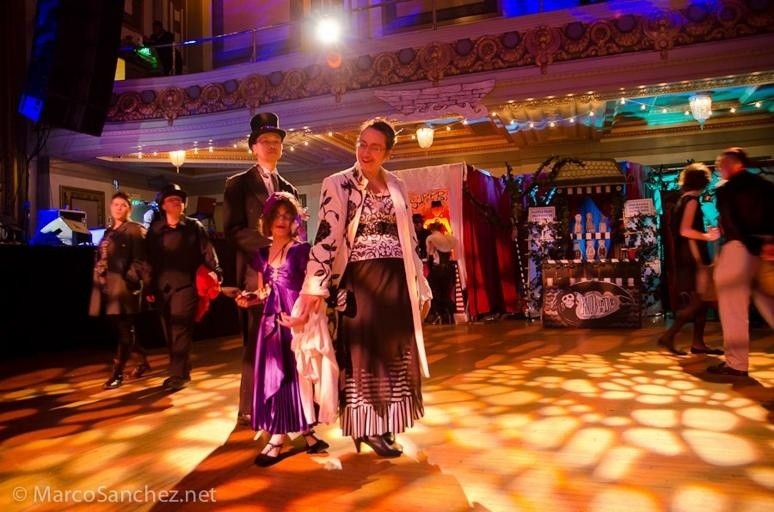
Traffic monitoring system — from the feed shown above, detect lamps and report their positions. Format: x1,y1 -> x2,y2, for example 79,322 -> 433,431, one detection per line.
416,127 -> 434,148
171,151 -> 185,173
688,94 -> 713,122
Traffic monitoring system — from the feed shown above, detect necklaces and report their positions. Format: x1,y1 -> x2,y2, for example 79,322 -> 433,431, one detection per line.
364,182 -> 387,213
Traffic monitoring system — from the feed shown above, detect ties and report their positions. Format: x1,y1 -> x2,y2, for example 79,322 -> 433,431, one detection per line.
271,174 -> 278,191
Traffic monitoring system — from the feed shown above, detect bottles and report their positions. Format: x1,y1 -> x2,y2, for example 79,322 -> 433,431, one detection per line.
573,214 -> 607,259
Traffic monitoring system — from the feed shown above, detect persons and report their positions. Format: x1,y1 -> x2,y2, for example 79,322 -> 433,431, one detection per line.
297,117 -> 433,454
236,191 -> 330,467
221,111 -> 299,425
655,162 -> 724,357
705,146 -> 774,377
411,213 -> 454,325
89,190 -> 152,390
142,183 -> 225,395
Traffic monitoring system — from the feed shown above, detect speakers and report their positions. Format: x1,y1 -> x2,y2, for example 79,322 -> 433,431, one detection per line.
18,0 -> 125,137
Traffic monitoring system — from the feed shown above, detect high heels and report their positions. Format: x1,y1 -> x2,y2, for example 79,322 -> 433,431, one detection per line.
446,314 -> 453,323
430,314 -> 442,325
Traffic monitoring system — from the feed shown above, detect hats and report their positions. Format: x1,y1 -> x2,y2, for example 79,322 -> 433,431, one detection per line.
249,113 -> 286,148
158,184 -> 187,210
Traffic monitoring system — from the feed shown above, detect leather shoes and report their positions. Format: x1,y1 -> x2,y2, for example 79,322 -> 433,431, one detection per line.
658,337 -> 687,355
103,363 -> 403,466
690,346 -> 724,355
707,362 -> 748,377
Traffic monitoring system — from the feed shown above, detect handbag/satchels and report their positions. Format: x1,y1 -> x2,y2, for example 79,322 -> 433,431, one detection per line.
698,264 -> 716,301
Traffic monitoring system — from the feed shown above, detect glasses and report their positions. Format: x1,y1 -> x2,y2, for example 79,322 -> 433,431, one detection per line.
357,142 -> 387,153
274,212 -> 294,222
